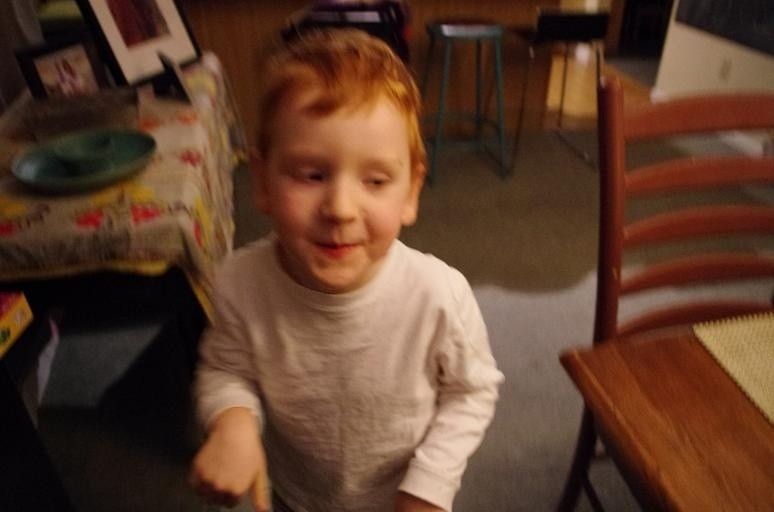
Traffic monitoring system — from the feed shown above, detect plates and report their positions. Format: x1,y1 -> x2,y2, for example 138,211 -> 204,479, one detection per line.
10,131 -> 158,196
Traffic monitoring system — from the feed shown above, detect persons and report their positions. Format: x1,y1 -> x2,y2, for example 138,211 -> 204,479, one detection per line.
191,29 -> 505,512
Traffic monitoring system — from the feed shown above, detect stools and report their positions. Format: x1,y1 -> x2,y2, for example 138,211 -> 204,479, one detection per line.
308,6 -> 391,40
416,19 -> 510,187
510,11 -> 609,177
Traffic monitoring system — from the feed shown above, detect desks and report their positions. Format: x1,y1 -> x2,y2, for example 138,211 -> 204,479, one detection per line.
0,44 -> 247,395
560,310 -> 774,512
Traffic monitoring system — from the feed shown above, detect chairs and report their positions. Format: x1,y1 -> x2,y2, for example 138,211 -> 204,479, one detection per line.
556,71 -> 774,512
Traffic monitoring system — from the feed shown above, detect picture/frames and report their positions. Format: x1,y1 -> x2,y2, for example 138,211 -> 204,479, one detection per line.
76,0 -> 202,90
13,32 -> 112,103
